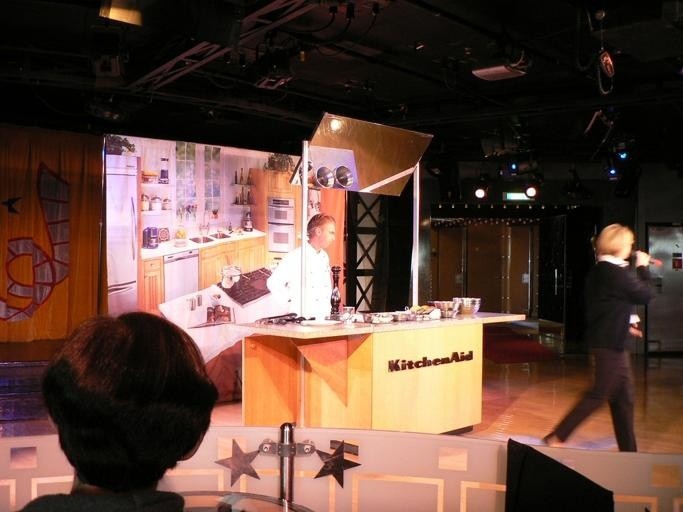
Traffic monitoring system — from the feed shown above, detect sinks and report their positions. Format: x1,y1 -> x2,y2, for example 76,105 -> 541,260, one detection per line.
189,235 -> 214,247
210,232 -> 232,240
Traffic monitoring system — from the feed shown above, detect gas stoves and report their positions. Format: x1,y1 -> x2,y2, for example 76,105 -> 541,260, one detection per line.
216,267 -> 273,310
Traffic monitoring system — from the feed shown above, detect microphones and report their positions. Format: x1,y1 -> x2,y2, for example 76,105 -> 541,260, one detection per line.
630,248 -> 662,270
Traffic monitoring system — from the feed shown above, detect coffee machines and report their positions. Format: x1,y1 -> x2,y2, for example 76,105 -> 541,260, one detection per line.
143,226 -> 158,249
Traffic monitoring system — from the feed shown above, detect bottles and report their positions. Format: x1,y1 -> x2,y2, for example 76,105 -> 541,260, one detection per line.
140,193 -> 172,211
235,187 -> 255,205
411,311 -> 429,321
190,298 -> 195,311
197,294 -> 202,306
159,157 -> 169,184
140,171 -> 158,184
233,167 -> 253,185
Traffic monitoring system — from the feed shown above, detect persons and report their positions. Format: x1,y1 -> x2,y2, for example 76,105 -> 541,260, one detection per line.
541,222 -> 652,451
266,213 -> 335,319
15,312 -> 219,512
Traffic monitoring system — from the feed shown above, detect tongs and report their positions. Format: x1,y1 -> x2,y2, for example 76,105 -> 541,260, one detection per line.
256,313 -> 297,326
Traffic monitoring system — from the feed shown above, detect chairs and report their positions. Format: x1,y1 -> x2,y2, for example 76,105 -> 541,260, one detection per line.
503,436 -> 616,512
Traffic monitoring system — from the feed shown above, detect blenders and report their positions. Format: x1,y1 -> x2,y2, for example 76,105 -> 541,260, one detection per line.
242,207 -> 253,232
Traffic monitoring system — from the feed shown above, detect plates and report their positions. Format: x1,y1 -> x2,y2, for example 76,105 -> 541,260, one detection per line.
172,241 -> 186,248
157,228 -> 169,241
299,320 -> 341,326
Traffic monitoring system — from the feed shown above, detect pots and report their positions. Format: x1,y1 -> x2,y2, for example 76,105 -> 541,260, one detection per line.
218,265 -> 242,293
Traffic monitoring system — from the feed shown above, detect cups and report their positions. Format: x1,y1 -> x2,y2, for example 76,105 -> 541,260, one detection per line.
343,307 -> 355,322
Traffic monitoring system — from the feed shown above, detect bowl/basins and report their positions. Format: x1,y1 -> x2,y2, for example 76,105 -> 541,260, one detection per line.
361,312 -> 410,323
428,297 -> 481,318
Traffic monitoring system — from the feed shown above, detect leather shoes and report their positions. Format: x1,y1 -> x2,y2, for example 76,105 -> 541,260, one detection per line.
540,431 -> 568,450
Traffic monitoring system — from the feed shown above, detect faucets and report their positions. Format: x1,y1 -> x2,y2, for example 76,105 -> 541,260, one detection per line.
199,209 -> 209,235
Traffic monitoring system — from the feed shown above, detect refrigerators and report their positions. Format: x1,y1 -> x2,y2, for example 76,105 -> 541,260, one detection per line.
106,154 -> 136,321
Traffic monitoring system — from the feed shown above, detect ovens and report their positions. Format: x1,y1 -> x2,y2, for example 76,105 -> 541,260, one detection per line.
266,196 -> 296,254
